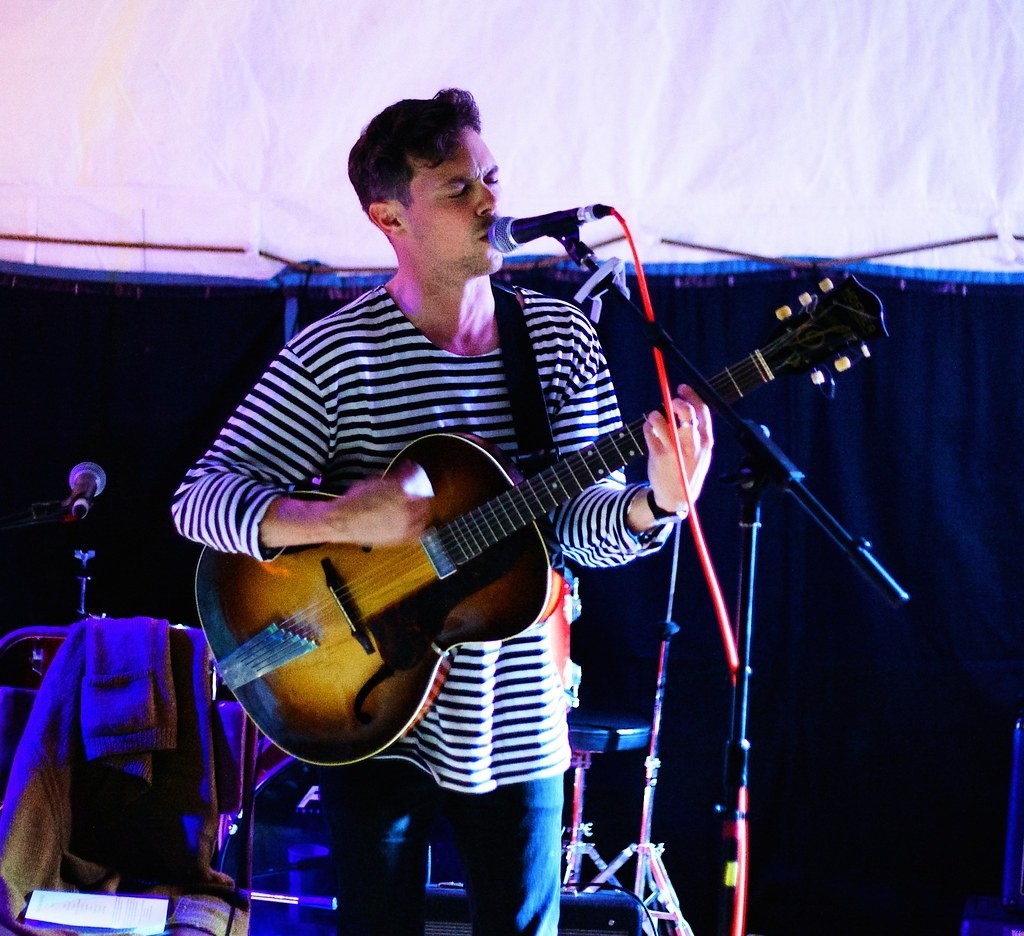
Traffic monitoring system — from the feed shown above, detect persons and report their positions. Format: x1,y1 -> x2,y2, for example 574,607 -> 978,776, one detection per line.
169,83 -> 716,936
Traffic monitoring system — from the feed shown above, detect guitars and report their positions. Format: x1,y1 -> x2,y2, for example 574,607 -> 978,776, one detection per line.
190,269 -> 899,771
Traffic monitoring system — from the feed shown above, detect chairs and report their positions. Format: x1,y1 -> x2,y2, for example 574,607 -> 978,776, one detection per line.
0,623 -> 258,935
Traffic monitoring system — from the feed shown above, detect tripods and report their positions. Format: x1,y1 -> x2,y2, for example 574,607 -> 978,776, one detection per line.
585,514 -> 694,936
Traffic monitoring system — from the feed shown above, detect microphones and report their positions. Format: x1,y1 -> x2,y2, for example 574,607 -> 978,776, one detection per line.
489,203 -> 612,253
68,462 -> 106,520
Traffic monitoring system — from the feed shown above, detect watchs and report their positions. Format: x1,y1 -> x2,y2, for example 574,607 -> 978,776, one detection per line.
645,489 -> 690,525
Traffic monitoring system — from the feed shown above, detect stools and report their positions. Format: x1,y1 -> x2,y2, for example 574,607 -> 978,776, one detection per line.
559,712 -> 652,894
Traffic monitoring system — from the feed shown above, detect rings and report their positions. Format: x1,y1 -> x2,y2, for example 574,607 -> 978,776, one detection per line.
679,419 -> 699,427
